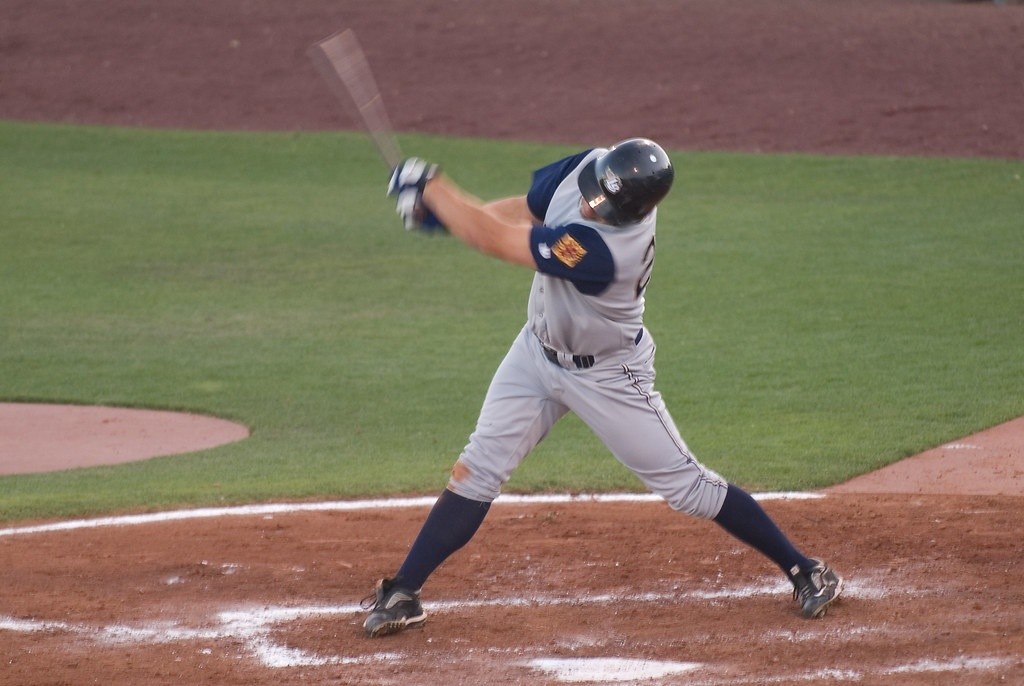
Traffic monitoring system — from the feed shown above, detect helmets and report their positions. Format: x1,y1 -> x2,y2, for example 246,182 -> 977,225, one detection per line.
578,138 -> 673,226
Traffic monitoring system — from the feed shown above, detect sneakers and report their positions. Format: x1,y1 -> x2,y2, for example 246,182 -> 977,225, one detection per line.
359,578 -> 426,636
792,556 -> 844,618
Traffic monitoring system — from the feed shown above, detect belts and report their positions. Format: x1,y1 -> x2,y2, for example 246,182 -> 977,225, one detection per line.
543,325 -> 644,369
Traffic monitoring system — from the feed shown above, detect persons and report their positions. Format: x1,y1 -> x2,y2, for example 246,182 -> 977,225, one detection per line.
360,137 -> 846,640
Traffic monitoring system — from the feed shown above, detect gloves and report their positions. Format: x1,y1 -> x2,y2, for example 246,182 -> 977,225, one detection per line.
396,185 -> 442,232
385,157 -> 440,194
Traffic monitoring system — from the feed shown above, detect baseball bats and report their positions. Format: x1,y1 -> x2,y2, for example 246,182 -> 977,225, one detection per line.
312,42 -> 399,169
